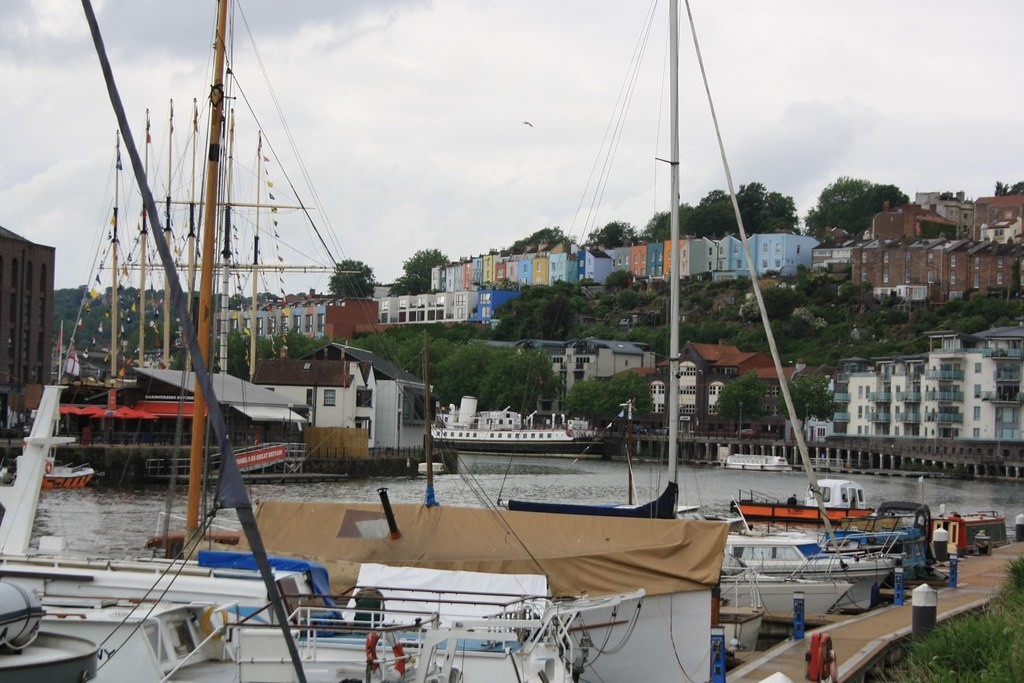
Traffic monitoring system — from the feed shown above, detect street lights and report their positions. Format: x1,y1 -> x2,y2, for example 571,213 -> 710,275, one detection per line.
739,399 -> 743,439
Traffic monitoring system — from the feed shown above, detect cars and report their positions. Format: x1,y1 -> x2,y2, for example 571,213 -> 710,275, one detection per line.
624,424 -> 648,435
735,428 -> 761,440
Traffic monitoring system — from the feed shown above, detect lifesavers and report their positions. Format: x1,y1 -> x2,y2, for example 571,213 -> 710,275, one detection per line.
45,463 -> 52,473
365,631 -> 405,683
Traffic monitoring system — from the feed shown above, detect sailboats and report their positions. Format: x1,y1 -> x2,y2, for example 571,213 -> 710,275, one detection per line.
0,1 -> 1010,682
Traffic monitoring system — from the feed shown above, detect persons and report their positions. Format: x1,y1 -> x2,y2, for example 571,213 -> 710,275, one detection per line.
786,493 -> 796,506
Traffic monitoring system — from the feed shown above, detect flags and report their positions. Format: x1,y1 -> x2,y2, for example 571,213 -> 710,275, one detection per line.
66,346 -> 80,378
56,326 -> 64,354
